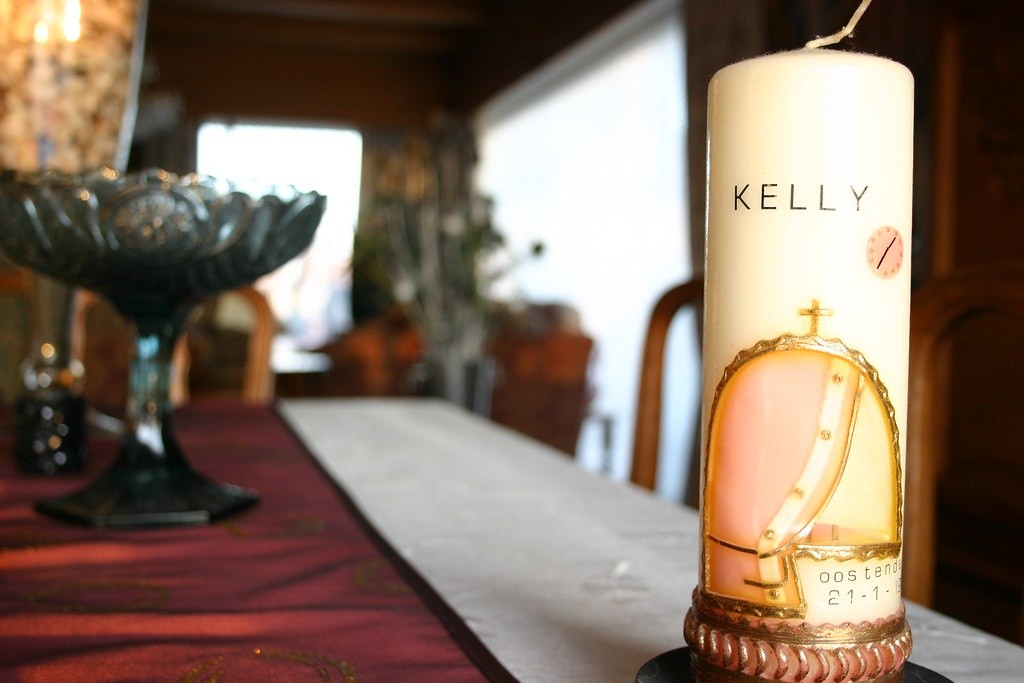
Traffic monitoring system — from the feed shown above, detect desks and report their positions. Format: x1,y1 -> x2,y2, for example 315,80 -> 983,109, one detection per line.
0,394 -> 1023,683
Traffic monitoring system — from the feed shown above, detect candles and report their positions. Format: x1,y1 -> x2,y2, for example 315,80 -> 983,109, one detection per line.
683,0 -> 913,683
